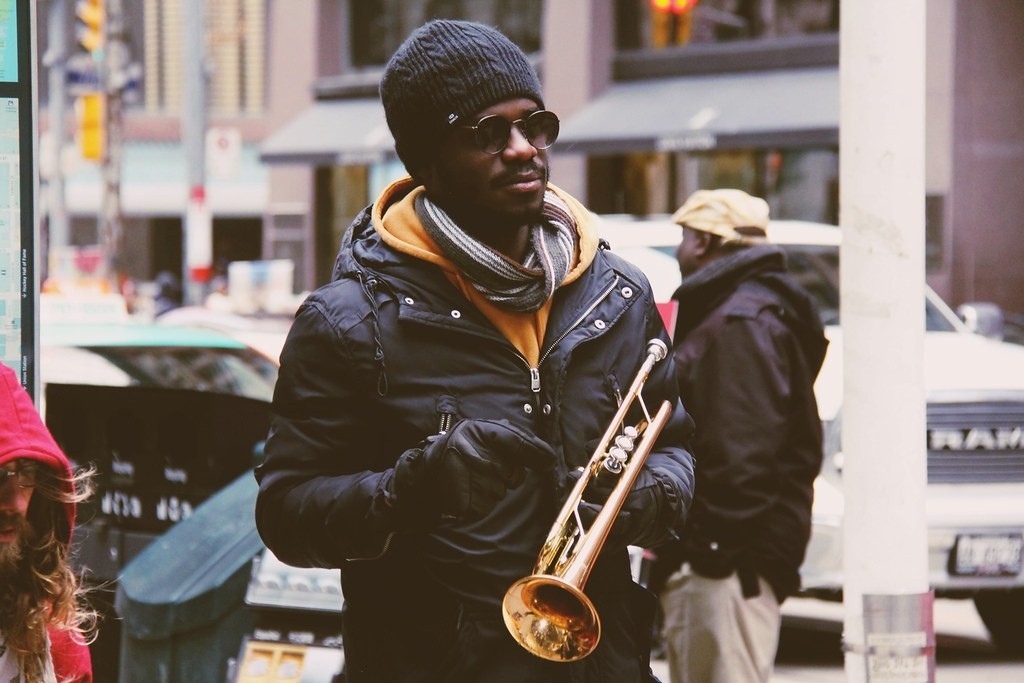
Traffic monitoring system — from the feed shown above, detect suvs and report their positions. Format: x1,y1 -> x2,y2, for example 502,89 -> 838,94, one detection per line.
607,210 -> 1023,654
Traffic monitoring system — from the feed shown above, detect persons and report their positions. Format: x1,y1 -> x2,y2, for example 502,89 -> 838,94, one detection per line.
657,188 -> 832,682
252,21 -> 702,682
0,361 -> 95,682
40,269 -> 232,329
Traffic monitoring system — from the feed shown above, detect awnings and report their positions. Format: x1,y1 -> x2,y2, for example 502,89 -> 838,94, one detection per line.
546,65 -> 880,152
253,74 -> 415,170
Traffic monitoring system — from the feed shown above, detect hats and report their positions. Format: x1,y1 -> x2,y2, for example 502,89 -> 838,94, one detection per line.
379,19 -> 545,182
669,188 -> 770,244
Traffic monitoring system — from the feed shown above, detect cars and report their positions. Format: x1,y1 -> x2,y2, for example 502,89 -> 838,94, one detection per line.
39,319 -> 282,410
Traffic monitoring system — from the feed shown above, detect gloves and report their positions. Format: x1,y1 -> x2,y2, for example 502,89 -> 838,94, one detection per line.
566,468 -> 663,558
390,418 -> 554,529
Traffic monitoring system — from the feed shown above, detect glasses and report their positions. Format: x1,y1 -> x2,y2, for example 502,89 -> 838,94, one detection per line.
458,110 -> 560,155
0,464 -> 43,488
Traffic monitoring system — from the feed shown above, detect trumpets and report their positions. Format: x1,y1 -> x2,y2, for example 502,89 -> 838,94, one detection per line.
498,335 -> 678,666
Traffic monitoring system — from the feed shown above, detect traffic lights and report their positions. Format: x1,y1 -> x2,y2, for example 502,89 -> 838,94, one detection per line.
73,0 -> 103,55
